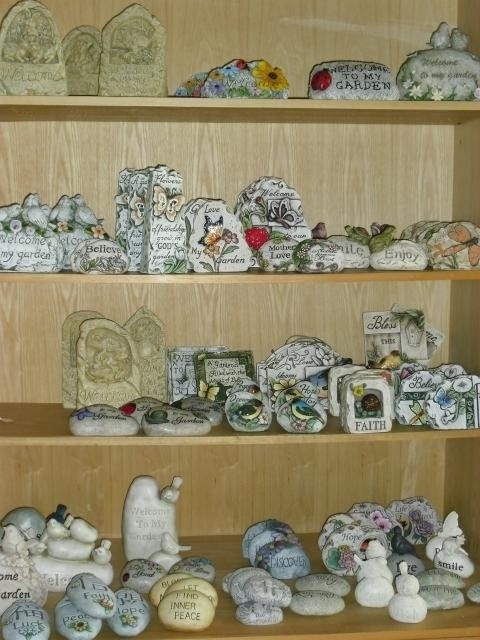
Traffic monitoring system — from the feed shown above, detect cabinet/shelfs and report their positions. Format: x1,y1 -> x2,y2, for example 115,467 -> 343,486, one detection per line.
0,0 -> 480,639
